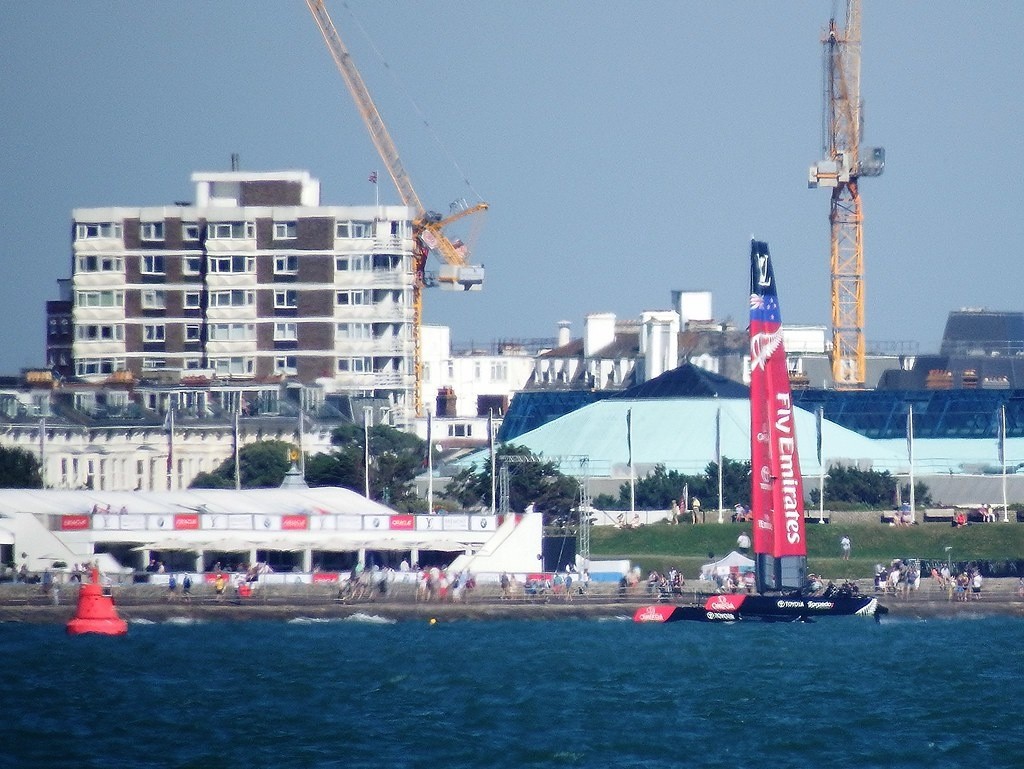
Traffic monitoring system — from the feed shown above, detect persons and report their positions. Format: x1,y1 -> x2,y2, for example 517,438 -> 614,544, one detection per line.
499,569 -> 592,601
619,561 -> 640,594
737,532 -> 751,556
666,500 -> 678,525
932,564 -> 982,601
894,513 -> 915,527
875,560 -> 920,598
91,504 -> 127,514
808,573 -> 824,596
979,504 -> 995,522
711,572 -> 755,591
145,557 -> 476,604
690,497 -> 701,524
841,535 -> 850,560
648,566 -> 683,602
733,503 -> 751,522
617,514 -> 639,528
5,552 -> 109,593
699,571 -> 705,580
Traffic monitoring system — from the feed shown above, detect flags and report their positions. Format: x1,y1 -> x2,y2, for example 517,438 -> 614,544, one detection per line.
369,171 -> 377,184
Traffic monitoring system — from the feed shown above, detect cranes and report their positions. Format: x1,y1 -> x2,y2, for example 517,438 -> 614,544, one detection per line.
304,0 -> 488,416
810,0 -> 887,389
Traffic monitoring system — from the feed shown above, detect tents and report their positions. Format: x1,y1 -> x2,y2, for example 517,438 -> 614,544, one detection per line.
703,551 -> 755,575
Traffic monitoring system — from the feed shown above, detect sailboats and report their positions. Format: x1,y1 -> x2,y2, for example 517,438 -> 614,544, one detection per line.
634,236 -> 890,624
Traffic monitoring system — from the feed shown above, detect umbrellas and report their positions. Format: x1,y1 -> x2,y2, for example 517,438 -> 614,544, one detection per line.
130,537 -> 482,552
